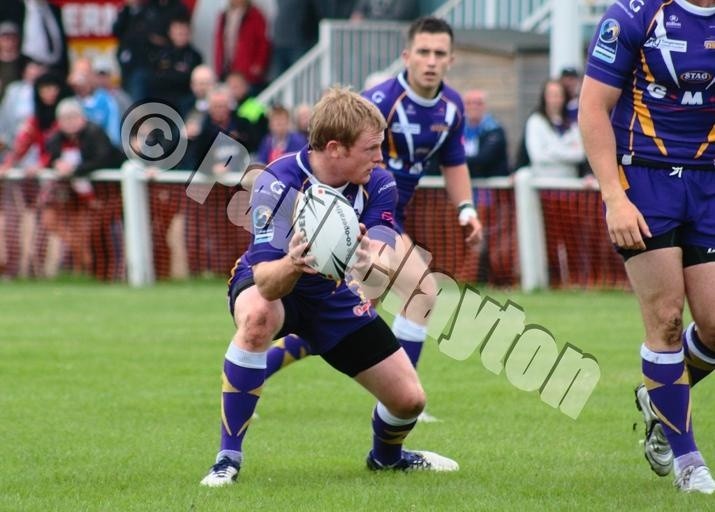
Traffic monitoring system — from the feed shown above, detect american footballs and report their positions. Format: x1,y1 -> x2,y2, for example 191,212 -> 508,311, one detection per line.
295,184 -> 363,283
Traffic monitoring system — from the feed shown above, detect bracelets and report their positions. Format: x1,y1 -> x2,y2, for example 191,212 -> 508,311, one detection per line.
457,201 -> 476,213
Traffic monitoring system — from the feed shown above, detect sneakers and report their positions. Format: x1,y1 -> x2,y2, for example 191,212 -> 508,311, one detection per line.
633,381 -> 675,476
367,448 -> 460,471
199,456 -> 240,488
672,465 -> 715,494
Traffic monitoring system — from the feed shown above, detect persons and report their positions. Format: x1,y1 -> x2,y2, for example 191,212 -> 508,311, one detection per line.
265,17 -> 485,422
200,82 -> 462,488
0,1 -> 316,212
457,68 -> 597,187
577,0 -> 715,497
